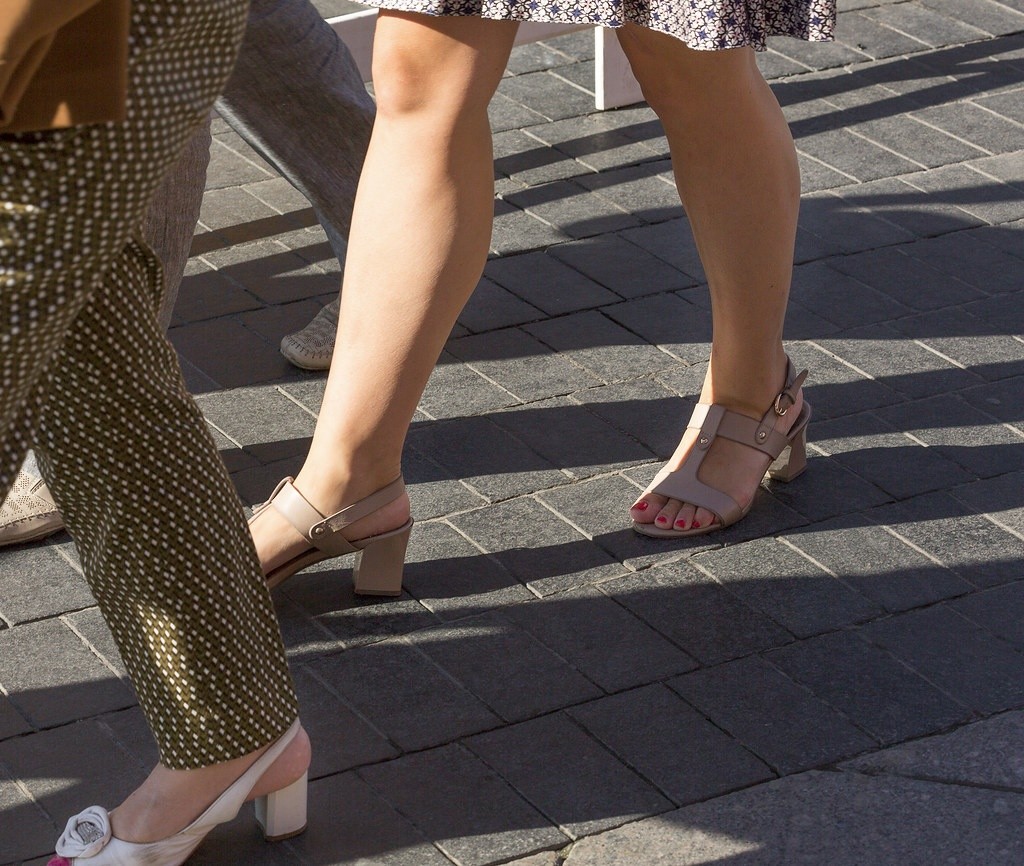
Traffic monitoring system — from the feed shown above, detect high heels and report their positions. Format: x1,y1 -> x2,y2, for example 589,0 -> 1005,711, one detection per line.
247,475 -> 413,597
49,716 -> 308,866
632,352 -> 811,538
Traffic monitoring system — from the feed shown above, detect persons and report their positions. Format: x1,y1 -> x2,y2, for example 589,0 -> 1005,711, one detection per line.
248,0 -> 839,596
0,0 -> 311,866
0,1 -> 379,547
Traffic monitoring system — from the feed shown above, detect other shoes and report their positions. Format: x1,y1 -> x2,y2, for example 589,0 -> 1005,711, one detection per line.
280,292 -> 340,370
0,450 -> 66,546
